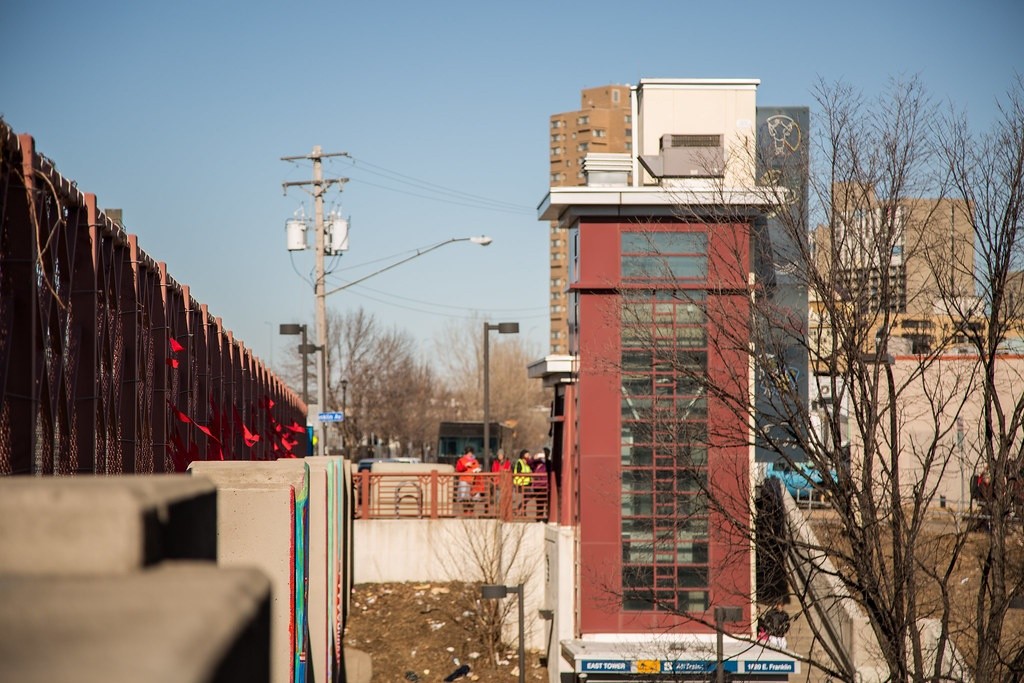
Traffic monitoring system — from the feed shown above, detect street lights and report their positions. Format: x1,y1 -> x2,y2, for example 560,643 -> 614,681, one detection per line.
481,321 -> 521,472
315,236 -> 493,451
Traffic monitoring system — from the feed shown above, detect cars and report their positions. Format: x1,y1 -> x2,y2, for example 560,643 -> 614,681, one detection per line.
768,462 -> 841,505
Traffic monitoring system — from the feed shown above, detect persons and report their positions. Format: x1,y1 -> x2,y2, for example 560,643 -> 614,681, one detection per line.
756,597 -> 792,650
455,446 -> 480,513
491,447 -> 555,523
